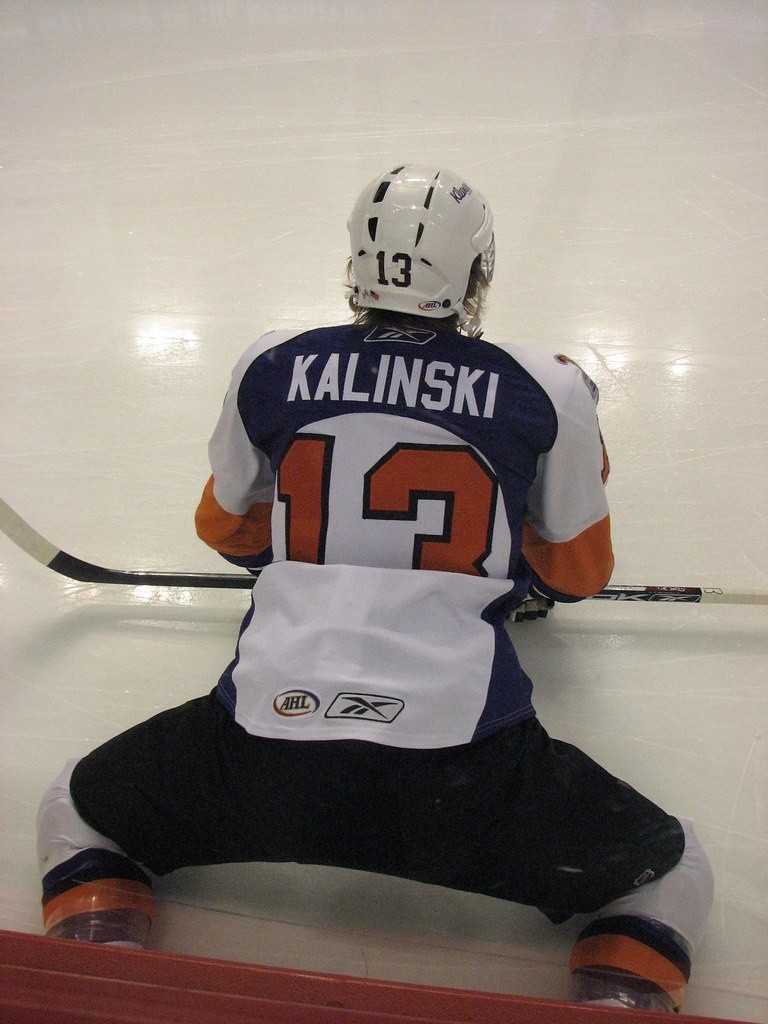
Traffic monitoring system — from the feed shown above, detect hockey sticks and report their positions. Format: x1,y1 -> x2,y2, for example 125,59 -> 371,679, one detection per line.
0,497 -> 767,612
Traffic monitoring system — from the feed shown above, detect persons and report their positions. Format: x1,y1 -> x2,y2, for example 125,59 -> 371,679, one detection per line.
36,165 -> 714,1014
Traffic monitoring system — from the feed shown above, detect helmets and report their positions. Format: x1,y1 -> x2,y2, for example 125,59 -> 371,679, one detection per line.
347,162 -> 495,317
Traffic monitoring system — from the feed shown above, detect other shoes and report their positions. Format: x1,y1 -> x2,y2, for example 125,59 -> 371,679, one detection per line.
575,965 -> 673,1015
43,909 -> 151,948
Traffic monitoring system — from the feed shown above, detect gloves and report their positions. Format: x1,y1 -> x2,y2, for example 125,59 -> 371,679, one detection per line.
505,583 -> 555,623
